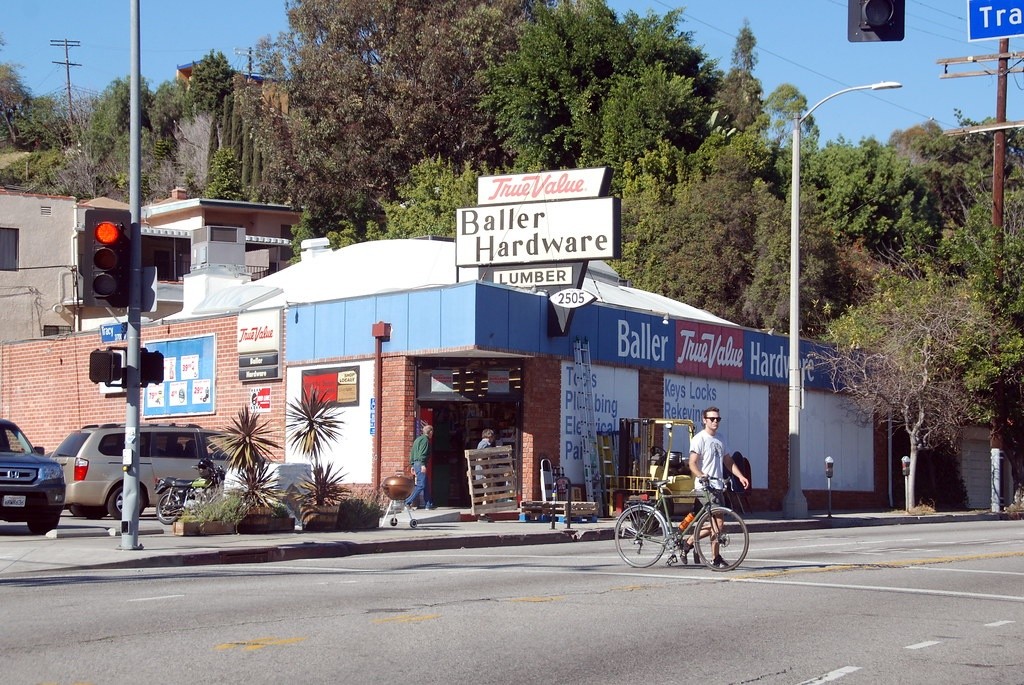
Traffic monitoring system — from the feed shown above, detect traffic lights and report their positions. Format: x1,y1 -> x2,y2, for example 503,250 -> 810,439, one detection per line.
844,0 -> 906,41
88,348 -> 122,383
83,207 -> 130,309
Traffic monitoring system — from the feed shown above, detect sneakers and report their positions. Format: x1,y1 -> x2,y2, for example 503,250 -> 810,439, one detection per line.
710,555 -> 735,570
477,516 -> 494,522
677,539 -> 694,564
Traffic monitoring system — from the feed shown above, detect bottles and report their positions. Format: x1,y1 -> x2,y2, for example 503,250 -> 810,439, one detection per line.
678,512 -> 695,532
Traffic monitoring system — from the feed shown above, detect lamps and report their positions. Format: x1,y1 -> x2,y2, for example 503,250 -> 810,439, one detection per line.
530,284 -> 538,293
158,318 -> 171,324
663,314 -> 670,324
284,301 -> 302,308
762,328 -> 774,337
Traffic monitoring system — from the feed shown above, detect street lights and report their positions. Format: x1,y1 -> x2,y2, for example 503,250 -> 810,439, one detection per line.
782,82 -> 905,519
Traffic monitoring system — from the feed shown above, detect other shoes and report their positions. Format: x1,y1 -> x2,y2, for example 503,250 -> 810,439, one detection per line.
404,502 -> 411,511
426,506 -> 437,510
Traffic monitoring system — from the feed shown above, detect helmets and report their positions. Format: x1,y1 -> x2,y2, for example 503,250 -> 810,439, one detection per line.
198,460 -> 208,468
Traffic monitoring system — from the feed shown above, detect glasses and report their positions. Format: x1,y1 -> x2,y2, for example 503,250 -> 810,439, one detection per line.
705,417 -> 722,422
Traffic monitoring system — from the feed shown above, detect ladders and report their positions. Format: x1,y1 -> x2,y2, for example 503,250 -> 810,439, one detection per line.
539,459 -> 557,502
573,337 -> 603,515
598,432 -> 619,517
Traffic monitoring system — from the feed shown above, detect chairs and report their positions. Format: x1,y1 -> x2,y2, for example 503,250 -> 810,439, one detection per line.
170,443 -> 184,455
185,439 -> 197,457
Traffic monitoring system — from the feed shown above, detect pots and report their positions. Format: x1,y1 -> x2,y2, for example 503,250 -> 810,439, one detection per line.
382,475 -> 413,500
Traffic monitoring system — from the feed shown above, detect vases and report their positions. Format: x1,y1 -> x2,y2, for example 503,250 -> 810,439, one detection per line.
302,511 -> 338,530
239,513 -> 295,532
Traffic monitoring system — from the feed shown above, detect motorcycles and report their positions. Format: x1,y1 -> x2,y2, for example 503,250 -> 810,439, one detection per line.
153,458 -> 224,526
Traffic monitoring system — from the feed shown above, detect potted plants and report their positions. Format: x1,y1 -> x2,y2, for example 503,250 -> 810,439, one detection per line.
173,491 -> 247,535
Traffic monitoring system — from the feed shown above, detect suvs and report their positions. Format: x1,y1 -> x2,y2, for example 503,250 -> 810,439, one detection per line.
0,420 -> 67,535
51,422 -> 236,520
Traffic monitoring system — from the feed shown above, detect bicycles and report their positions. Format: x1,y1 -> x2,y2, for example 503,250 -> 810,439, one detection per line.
614,476 -> 749,572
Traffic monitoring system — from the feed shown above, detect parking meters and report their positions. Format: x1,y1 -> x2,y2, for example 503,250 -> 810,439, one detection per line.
825,456 -> 836,517
901,456 -> 911,512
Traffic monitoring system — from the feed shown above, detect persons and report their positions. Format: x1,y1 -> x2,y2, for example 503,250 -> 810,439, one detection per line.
679,407 -> 748,569
476,429 -> 499,505
404,425 -> 438,510
